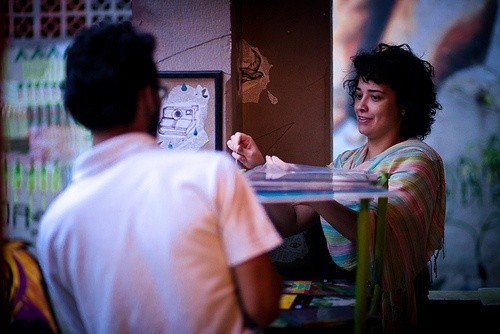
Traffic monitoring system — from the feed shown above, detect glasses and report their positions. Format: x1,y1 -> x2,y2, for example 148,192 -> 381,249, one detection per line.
153,84 -> 168,99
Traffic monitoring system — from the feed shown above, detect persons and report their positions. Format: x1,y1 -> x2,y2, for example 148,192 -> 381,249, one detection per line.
225,44 -> 447,333
34,22 -> 283,333
